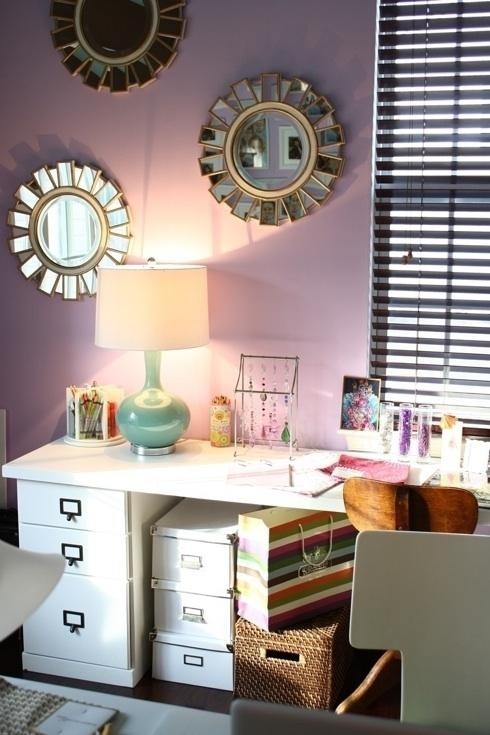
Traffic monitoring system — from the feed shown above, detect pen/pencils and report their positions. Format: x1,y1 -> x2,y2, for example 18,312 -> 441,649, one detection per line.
69,377 -> 104,441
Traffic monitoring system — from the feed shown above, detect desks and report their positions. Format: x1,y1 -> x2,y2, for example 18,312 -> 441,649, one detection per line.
3,437 -> 442,690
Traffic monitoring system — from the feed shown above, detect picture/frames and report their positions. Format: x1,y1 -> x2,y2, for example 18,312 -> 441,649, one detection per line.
236,118 -> 270,170
278,126 -> 302,170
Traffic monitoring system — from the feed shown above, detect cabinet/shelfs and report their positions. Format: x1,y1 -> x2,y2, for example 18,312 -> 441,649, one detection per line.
16,479 -> 136,670
149,499 -> 263,698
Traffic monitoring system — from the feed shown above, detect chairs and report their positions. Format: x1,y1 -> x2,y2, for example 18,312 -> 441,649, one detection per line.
335,478 -> 474,716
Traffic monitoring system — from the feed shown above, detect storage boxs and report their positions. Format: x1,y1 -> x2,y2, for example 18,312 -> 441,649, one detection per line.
232,617 -> 340,712
236,508 -> 358,630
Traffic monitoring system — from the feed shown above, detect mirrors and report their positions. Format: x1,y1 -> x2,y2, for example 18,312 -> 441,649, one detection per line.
199,71 -> 343,227
47,1 -> 187,93
9,163 -> 132,300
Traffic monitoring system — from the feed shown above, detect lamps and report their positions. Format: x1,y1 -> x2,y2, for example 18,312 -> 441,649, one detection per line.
95,256 -> 209,457
